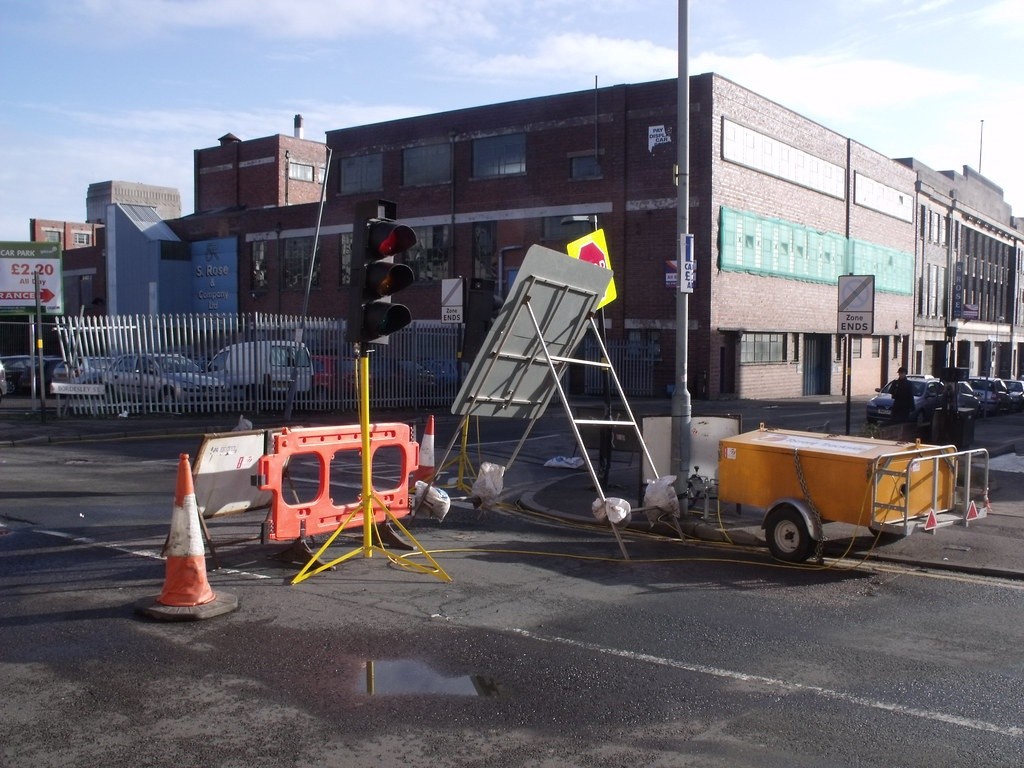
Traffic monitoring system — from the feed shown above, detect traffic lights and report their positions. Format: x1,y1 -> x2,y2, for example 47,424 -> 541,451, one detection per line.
361,218 -> 418,341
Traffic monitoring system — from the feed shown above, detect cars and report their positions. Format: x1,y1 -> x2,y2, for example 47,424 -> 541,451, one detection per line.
1002,380 -> 1024,409
52,356 -> 115,397
311,354 -> 460,410
968,377 -> 1013,415
866,374 -> 948,428
104,353 -> 232,412
0,355 -> 63,398
955,381 -> 984,418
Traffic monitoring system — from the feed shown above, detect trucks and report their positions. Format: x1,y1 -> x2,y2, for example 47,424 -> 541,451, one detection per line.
205,340 -> 313,400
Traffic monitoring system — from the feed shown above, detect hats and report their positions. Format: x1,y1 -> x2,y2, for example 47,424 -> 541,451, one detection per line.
897,367 -> 906,373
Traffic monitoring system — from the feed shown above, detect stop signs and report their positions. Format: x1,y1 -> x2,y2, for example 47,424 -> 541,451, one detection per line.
567,227 -> 617,311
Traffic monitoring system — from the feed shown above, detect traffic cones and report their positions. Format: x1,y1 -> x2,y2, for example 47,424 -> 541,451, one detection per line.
407,414 -> 435,493
132,453 -> 238,621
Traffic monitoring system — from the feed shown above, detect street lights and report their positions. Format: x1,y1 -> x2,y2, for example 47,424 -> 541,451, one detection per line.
560,214 -> 611,421
996,315 -> 1004,344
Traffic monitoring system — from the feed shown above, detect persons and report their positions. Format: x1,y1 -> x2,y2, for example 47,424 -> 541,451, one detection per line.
891,367 -> 915,441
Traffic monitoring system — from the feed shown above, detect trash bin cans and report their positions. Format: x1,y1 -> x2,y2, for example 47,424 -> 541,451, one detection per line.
933,407 -> 975,447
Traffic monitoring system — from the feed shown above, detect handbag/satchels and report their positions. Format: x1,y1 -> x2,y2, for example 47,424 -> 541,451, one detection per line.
892,380 -> 899,399
